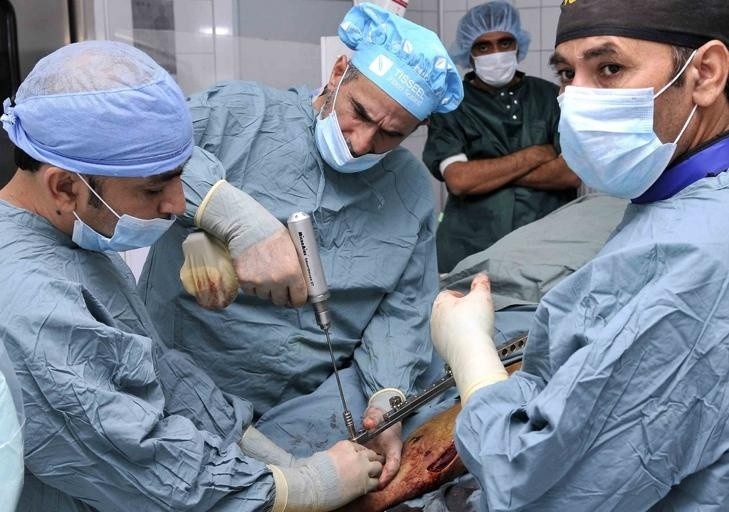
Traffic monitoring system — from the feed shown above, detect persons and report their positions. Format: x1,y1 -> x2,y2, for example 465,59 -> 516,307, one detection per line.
423,0 -> 584,277
431,1 -> 729,512
0,40 -> 386,512
133,4 -> 463,495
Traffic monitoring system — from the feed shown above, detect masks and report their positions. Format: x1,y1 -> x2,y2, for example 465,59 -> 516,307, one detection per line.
469,45 -> 519,87
314,68 -> 393,174
71,175 -> 176,254
556,51 -> 698,200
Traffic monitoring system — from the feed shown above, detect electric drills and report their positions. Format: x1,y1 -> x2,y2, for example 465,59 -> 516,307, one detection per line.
287,211 -> 356,438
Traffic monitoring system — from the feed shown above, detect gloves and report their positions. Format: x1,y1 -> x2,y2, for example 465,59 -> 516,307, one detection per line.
430,274 -> 510,407
193,180 -> 307,306
363,387 -> 407,487
241,425 -> 384,512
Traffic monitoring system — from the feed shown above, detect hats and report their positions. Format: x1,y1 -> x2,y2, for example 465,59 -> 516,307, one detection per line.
338,2 -> 465,122
0,40 -> 194,177
